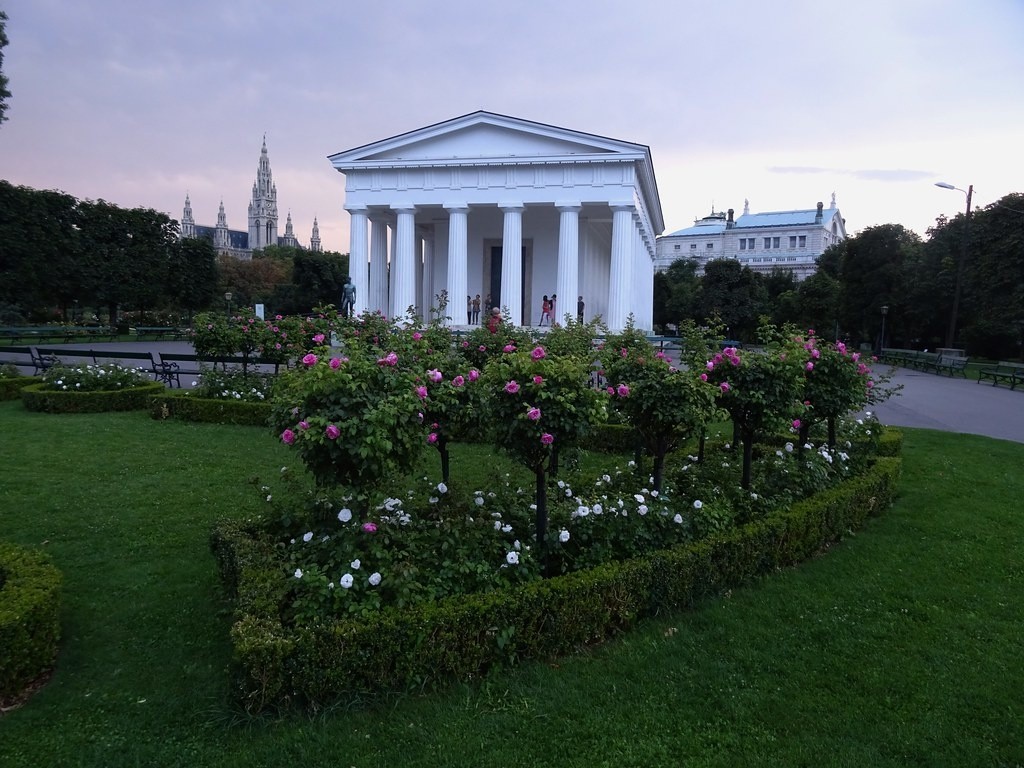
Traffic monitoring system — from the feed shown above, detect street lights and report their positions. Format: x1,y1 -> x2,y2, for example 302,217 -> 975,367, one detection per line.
935,181 -> 976,349
226,292 -> 232,320
880,306 -> 888,355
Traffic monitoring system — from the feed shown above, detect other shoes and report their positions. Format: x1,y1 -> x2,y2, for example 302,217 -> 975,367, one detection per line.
538,325 -> 541,326
476,323 -> 477,325
472,323 -> 474,325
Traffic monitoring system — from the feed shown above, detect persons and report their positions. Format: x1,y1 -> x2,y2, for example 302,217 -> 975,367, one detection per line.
342,276 -> 357,319
467,293 -> 504,335
538,294 -> 585,326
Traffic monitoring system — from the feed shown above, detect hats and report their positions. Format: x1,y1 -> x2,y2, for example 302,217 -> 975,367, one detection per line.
551,294 -> 556,298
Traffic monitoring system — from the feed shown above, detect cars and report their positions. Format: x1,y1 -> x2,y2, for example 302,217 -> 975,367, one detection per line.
718,340 -> 743,350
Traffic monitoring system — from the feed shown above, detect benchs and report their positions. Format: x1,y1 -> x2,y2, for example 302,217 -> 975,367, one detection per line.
977,361 -> 1024,391
876,348 -> 970,379
0,326 -> 189,341
375,328 -> 743,363
213,356 -> 294,385
90,349 -> 169,385
34,347 -> 95,379
0,346 -> 39,376
157,352 -> 216,393
